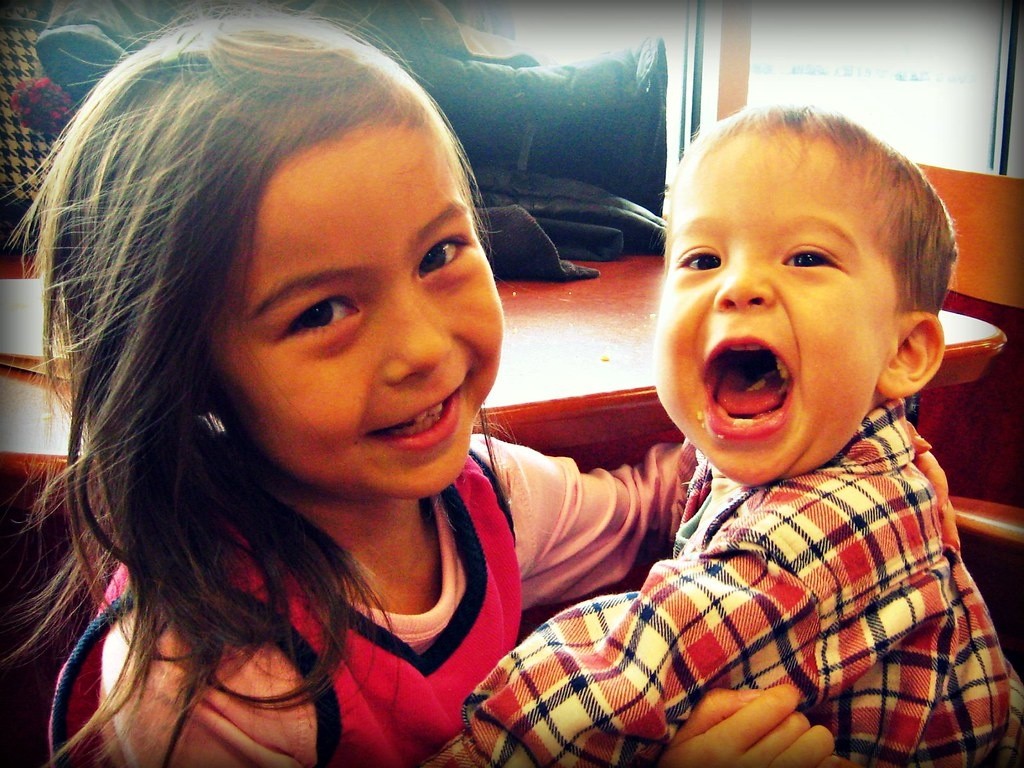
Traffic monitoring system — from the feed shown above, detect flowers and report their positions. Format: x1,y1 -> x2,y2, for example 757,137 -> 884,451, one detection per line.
11,77 -> 78,137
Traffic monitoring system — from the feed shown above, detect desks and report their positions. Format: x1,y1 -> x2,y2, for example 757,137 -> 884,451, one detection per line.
1,254 -> 1008,587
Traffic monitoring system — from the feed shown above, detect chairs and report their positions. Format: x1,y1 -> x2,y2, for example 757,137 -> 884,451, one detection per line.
0,0 -> 86,209
908,162 -> 1024,549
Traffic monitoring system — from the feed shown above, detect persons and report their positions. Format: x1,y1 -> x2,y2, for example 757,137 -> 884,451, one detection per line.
420,102 -> 1024,768
29,16 -> 960,768
